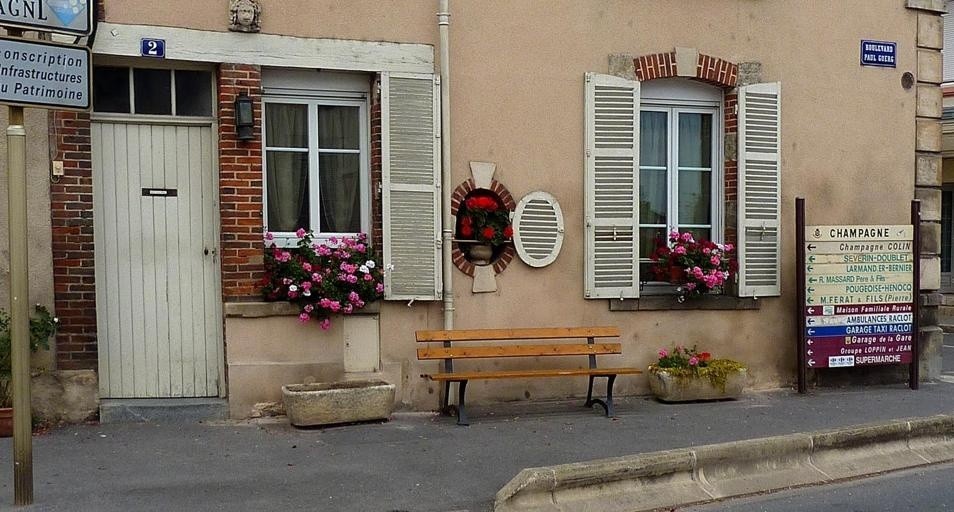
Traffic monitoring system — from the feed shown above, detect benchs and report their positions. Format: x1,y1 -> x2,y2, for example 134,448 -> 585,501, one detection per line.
414,326 -> 642,426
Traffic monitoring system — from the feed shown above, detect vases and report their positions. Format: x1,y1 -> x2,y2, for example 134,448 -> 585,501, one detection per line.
646,359 -> 748,403
0,408 -> 13,437
470,244 -> 493,265
281,378 -> 396,429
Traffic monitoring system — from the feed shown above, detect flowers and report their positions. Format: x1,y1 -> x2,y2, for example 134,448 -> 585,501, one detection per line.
0,303 -> 60,408
255,227 -> 385,330
458,196 -> 515,246
654,342 -> 712,368
648,227 -> 738,301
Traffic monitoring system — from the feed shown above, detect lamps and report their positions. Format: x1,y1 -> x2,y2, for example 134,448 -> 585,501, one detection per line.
234,89 -> 257,142
